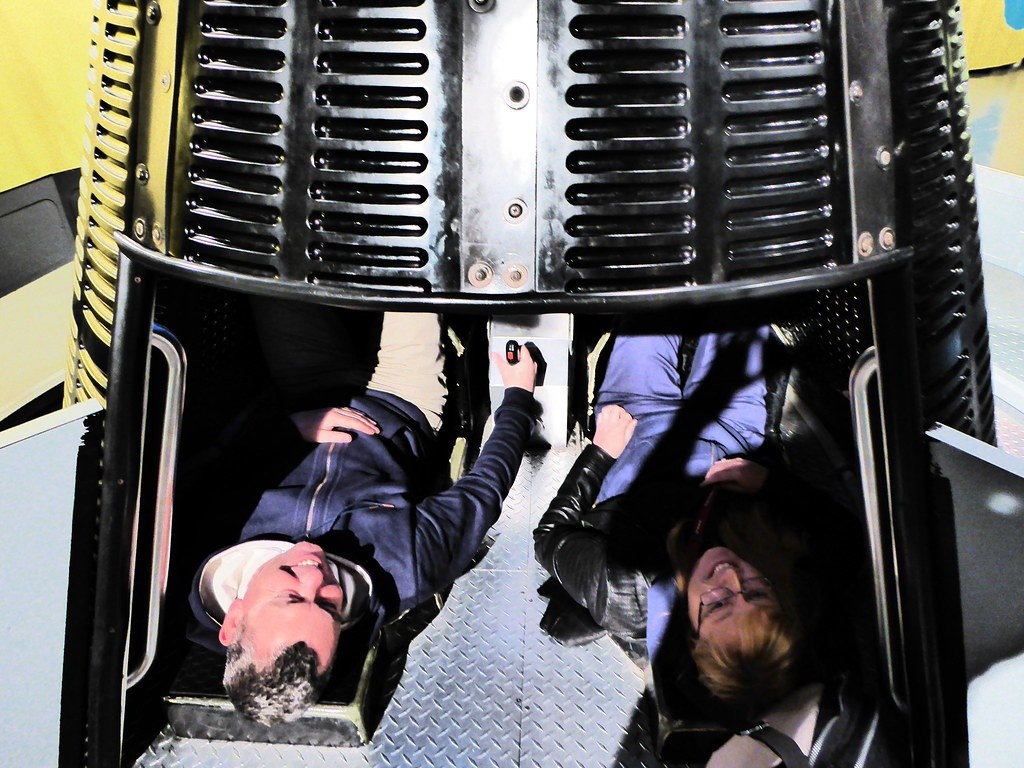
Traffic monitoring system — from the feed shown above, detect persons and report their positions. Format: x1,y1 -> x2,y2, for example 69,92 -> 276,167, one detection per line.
181,305 -> 539,728
532,327 -> 802,700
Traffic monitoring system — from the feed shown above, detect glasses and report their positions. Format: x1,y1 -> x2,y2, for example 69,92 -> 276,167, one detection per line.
698,576 -> 775,637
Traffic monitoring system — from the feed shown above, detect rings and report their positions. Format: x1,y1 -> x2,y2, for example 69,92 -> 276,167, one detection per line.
721,459 -> 726,464
